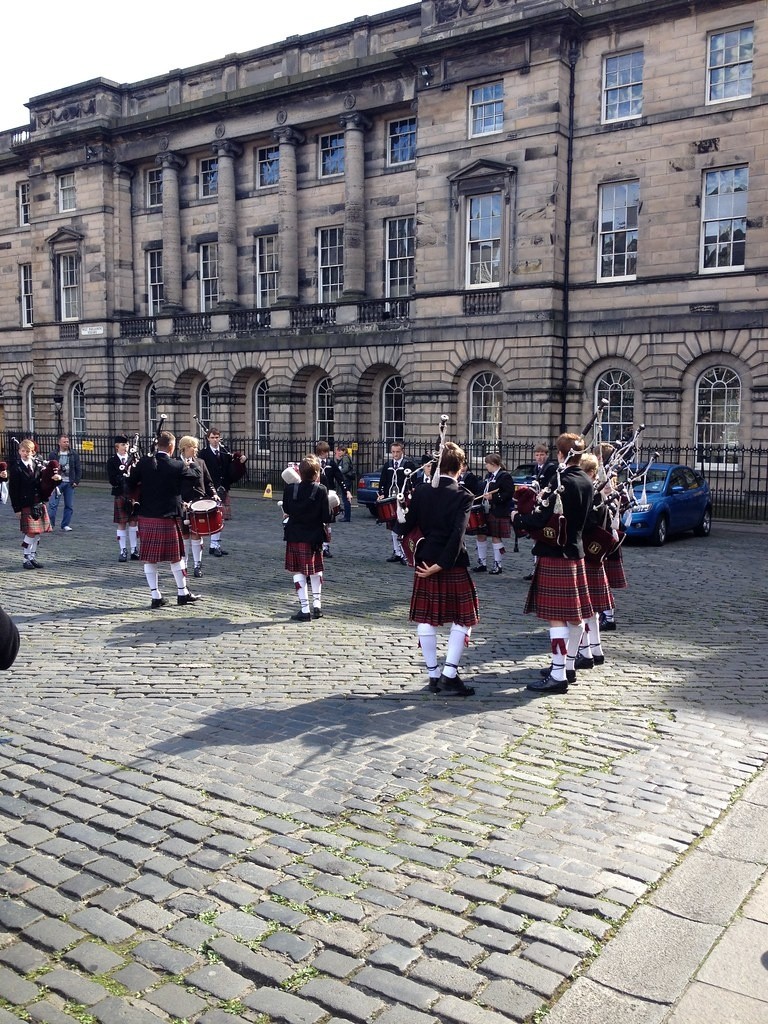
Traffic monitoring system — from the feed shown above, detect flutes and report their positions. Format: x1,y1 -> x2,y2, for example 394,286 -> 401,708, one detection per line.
395,413 -> 450,516
118,412 -> 168,478
615,451 -> 660,515
532,397 -> 610,515
9,436 -> 49,472
192,414 -> 241,463
592,422 -> 648,501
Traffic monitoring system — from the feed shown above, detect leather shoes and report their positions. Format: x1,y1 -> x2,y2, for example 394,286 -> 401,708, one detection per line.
429,673 -> 475,695
540,666 -> 577,682
526,674 -> 569,693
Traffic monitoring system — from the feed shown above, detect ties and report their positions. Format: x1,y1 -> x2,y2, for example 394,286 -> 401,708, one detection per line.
488,474 -> 494,482
394,462 -> 398,470
537,466 -> 541,476
214,450 -> 219,459
321,460 -> 326,469
122,458 -> 127,467
424,478 -> 430,483
26,464 -> 33,476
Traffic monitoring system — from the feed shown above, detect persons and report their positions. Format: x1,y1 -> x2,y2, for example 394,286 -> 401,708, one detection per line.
8,440 -> 61,569
406,440 -> 481,696
47,435 -> 82,531
282,440 -> 354,622
378,442 -> 515,576
107,429 -> 246,578
129,430 -> 202,608
511,433 -> 628,693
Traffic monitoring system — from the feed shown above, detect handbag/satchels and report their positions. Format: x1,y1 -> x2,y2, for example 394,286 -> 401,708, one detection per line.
341,455 -> 357,481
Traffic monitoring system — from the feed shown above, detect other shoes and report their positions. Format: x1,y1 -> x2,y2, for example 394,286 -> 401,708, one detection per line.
574,653 -> 605,669
60,526 -> 72,532
523,572 -> 534,581
291,610 -> 311,622
337,517 -> 351,522
489,560 -> 503,575
22,559 -> 44,569
386,553 -> 407,566
118,545 -> 229,609
312,607 -> 323,619
322,549 -> 333,558
599,612 -> 617,631
470,558 -> 487,572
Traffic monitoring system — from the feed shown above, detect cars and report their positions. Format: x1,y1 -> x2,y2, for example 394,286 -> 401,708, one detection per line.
356,457 -> 422,514
617,462 -> 712,547
509,460 -> 557,492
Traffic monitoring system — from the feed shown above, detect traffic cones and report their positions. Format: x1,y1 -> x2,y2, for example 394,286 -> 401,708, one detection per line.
263,484 -> 273,498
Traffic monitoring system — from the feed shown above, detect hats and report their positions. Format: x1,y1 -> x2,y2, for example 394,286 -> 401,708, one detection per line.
114,434 -> 129,443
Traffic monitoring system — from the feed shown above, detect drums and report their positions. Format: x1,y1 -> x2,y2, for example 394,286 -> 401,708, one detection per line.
187,496 -> 224,536
374,496 -> 406,522
464,503 -> 488,534
332,503 -> 342,517
